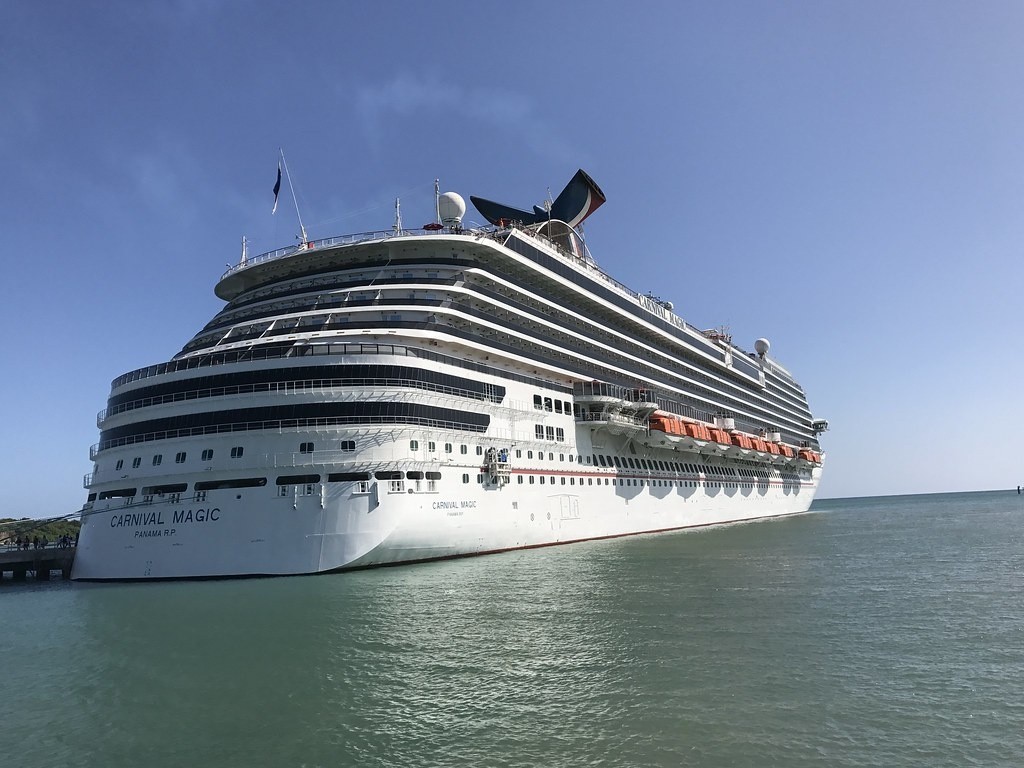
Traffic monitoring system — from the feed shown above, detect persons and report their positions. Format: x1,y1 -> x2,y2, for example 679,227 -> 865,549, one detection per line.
450,225 -> 463,235
15,533 -> 79,552
500,218 -> 516,230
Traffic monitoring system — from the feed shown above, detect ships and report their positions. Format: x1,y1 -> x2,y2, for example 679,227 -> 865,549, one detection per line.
69,146 -> 830,580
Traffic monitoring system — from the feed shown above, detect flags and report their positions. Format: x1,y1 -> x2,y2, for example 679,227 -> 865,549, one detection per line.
272,160 -> 282,214
